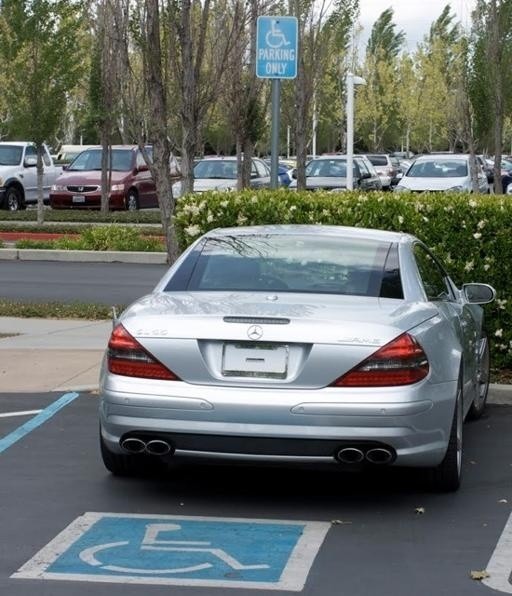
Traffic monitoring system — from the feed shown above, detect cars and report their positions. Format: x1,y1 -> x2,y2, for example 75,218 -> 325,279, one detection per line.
98,223 -> 498,493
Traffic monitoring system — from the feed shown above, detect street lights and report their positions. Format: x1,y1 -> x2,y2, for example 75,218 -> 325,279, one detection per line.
345,71 -> 368,193
